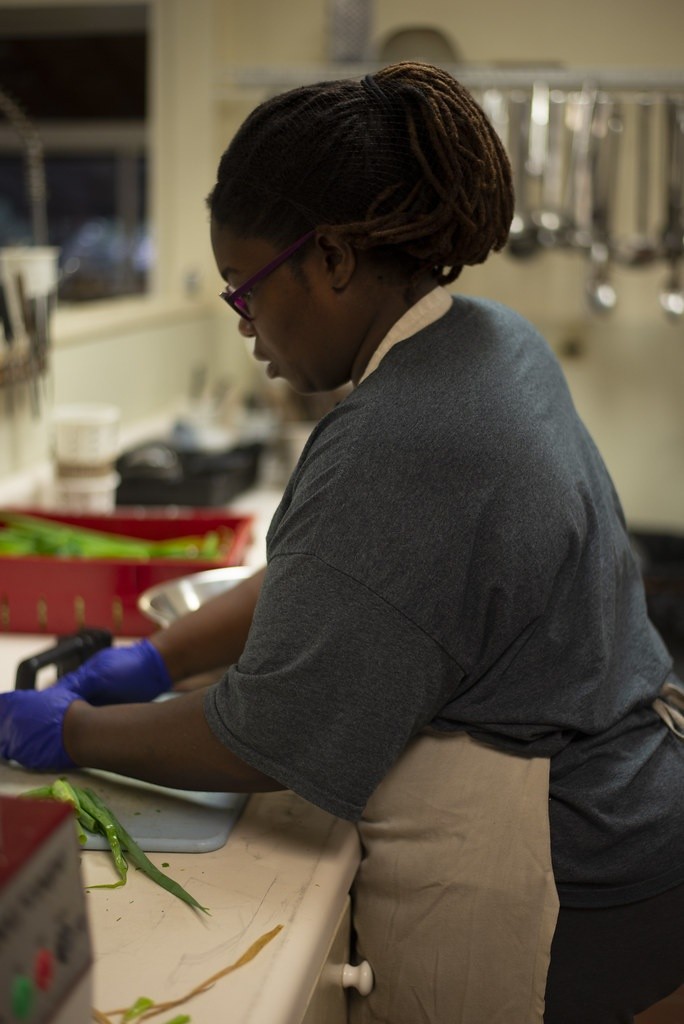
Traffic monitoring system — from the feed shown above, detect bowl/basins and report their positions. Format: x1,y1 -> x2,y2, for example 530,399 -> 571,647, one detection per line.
137,566 -> 261,630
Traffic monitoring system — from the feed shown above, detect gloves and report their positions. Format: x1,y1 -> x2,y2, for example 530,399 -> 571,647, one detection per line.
0,689 -> 90,775
57,637 -> 173,706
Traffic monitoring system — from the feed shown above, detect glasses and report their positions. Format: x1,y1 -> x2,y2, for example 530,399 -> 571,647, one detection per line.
217,224 -> 326,321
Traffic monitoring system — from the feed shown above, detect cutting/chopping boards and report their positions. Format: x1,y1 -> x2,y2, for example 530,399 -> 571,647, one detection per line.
0,691 -> 249,854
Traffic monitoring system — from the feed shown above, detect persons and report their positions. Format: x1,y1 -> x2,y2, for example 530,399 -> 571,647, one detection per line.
0,62 -> 684,1024
